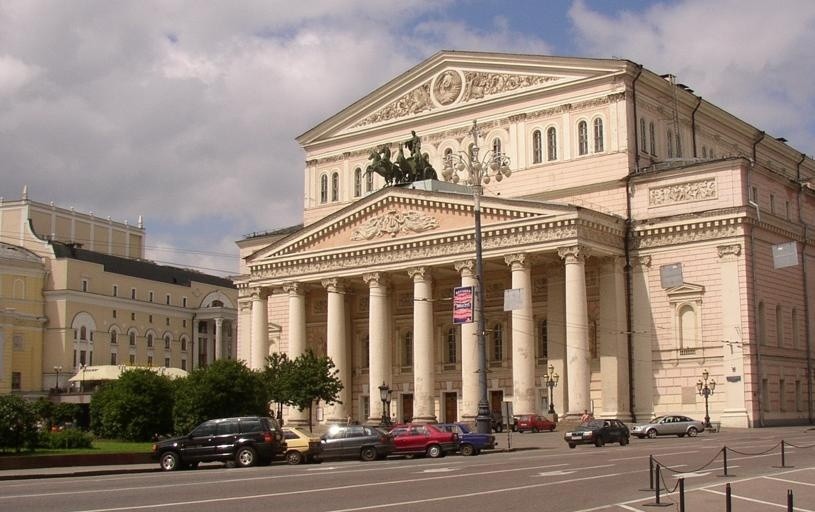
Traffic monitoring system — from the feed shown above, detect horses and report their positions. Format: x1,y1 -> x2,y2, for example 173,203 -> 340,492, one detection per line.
391,143 -> 413,182
371,146 -> 402,185
414,142 -> 425,182
362,151 -> 394,188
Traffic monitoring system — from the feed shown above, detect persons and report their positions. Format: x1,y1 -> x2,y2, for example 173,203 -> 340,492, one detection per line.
581,410 -> 590,424
378,144 -> 391,166
347,414 -> 353,427
409,131 -> 421,156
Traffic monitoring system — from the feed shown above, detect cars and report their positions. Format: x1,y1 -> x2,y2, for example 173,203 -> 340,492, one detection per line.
517,414 -> 558,435
491,417 -> 520,433
382,420 -> 461,460
314,423 -> 393,463
269,424 -> 325,466
630,414 -> 706,440
562,417 -> 632,450
436,422 -> 498,458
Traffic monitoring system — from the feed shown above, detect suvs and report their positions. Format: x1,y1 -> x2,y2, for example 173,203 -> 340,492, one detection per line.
147,413 -> 287,472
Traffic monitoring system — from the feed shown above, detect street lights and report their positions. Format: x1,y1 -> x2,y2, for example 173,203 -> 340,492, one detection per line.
543,363 -> 561,414
438,117 -> 516,433
386,388 -> 395,425
118,363 -> 128,374
79,361 -> 89,392
696,368 -> 718,430
378,380 -> 390,425
52,365 -> 64,393
